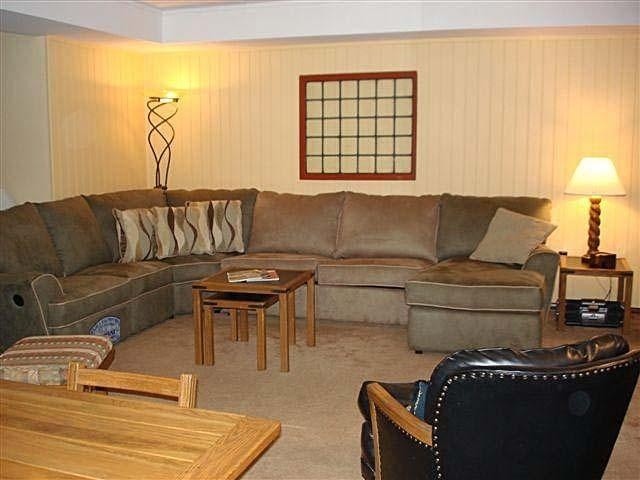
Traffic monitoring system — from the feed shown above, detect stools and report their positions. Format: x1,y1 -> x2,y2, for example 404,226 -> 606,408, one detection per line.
0,335 -> 116,399
201,293 -> 296,371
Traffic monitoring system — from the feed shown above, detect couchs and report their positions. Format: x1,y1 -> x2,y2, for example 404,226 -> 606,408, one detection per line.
0,188 -> 560,357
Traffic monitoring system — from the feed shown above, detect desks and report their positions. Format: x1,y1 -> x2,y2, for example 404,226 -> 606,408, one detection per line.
0,379 -> 283,478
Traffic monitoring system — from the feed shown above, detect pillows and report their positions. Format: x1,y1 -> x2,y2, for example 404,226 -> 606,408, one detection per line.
469,204 -> 558,268
112,197 -> 248,264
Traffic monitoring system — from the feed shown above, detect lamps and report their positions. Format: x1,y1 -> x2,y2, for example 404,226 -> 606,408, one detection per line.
564,157 -> 627,262
144,94 -> 179,187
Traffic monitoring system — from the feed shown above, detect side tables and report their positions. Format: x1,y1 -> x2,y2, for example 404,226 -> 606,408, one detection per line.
553,254 -> 635,337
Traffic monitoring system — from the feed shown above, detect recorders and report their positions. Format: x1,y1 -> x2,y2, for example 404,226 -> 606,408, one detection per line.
566,299 -> 624,327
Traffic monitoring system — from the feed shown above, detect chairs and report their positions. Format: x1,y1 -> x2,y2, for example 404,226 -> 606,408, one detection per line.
65,360 -> 198,411
356,335 -> 640,479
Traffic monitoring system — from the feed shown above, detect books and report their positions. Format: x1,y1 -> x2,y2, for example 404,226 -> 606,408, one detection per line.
247,269 -> 279,282
226,268 -> 265,283
